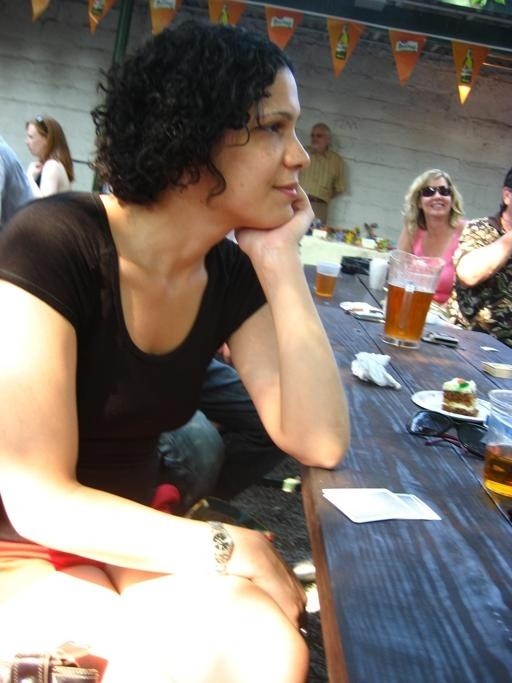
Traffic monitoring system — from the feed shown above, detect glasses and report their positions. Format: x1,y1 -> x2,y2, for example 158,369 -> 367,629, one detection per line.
420,185 -> 452,197
35,115 -> 48,133
405,410 -> 488,459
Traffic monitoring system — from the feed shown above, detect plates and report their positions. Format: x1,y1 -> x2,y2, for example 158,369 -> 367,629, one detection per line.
340,299 -> 383,325
411,386 -> 486,427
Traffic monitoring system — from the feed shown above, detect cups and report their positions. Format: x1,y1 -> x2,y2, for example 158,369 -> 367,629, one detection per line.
314,260 -> 342,302
362,238 -> 376,249
369,258 -> 388,291
383,250 -> 445,350
484,388 -> 511,500
312,229 -> 327,238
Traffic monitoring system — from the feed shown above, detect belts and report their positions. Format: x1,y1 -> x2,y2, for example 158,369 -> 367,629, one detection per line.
307,196 -> 326,202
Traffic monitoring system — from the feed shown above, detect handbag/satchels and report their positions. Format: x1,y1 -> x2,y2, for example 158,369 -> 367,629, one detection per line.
341,256 -> 372,274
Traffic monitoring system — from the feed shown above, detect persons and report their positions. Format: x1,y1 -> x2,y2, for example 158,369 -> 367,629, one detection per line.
296,121 -> 347,228
396,169 -> 470,308
0,134 -> 39,229
25,112 -> 75,198
0,15 -> 351,683
451,165 -> 512,351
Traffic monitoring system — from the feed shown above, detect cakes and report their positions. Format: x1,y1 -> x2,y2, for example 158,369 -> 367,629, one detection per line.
441,377 -> 480,417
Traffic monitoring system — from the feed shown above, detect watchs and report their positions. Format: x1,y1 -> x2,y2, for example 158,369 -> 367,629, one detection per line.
206,520 -> 234,575
